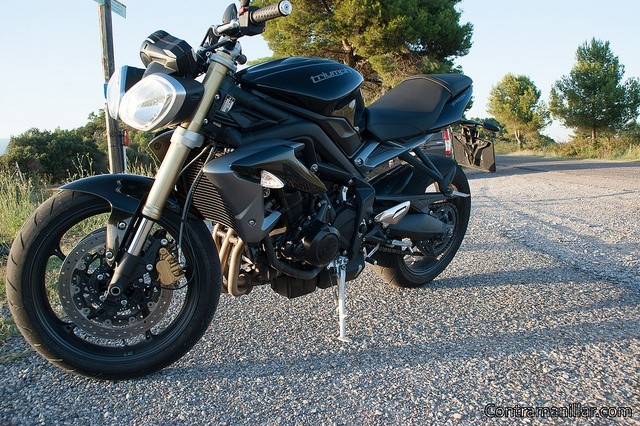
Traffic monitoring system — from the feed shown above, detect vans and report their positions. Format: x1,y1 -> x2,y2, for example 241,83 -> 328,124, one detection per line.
389,129 -> 452,166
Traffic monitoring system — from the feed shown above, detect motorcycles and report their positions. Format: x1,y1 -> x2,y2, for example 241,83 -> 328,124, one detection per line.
5,0 -> 499,381
